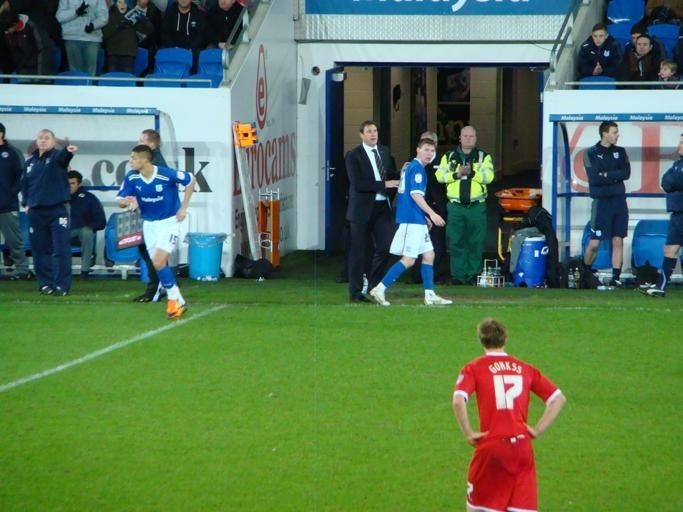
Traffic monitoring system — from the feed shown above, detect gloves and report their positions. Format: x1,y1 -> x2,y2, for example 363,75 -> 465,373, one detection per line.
84,22 -> 93,33
76,0 -> 90,16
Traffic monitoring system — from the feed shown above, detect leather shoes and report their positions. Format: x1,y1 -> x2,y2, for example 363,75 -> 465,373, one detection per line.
335,274 -> 348,283
349,294 -> 371,304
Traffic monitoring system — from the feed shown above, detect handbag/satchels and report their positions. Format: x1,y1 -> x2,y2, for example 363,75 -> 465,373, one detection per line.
234,253 -> 276,279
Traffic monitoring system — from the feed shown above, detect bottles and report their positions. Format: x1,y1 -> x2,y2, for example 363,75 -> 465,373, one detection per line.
567,267 -> 574,289
477,267 -> 501,289
573,268 -> 580,289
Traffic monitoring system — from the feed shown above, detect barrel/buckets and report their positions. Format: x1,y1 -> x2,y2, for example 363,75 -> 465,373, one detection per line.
509,233 -> 550,290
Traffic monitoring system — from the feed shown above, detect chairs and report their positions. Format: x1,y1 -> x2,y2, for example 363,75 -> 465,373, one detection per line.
587,216 -> 683,287
0,207 -> 180,282
567,0 -> 683,94
0,0 -> 259,90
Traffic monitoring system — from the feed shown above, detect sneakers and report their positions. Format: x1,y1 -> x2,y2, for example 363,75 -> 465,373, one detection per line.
80,271 -> 88,281
52,288 -> 68,296
160,280 -> 179,297
133,292 -> 161,302
636,283 -> 664,297
609,279 -> 625,288
166,299 -> 180,318
369,287 -> 390,306
424,295 -> 452,305
166,304 -> 187,319
582,270 -> 592,288
8,270 -> 35,281
39,286 -> 55,295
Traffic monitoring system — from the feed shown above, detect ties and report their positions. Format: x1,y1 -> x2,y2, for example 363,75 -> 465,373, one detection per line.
370,149 -> 386,181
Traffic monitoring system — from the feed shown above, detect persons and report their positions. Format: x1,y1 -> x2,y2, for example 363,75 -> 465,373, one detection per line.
578,1 -> 682,91
637,133 -> 683,301
0,0 -> 247,85
583,117 -> 631,288
345,121 -> 493,306
450,318 -> 566,511
0,123 -> 198,321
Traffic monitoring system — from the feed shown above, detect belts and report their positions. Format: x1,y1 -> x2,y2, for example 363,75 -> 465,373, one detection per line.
453,200 -> 479,207
